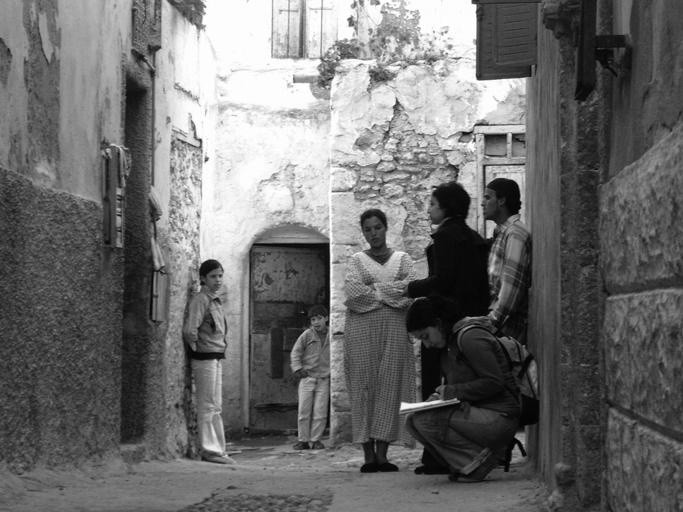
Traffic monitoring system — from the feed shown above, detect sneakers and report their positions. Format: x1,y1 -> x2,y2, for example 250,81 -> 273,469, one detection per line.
448,457 -> 498,483
292,441 -> 325,450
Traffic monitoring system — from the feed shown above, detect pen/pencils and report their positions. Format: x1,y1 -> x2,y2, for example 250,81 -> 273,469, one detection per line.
442,377 -> 444,386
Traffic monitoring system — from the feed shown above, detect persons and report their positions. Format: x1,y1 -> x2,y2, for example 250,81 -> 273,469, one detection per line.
406,183 -> 490,474
481,182 -> 533,466
183,260 -> 236,464
342,208 -> 417,472
290,305 -> 330,450
404,298 -> 523,483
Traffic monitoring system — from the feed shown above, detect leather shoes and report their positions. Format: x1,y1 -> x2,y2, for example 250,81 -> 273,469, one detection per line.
201,450 -> 236,464
360,462 -> 399,473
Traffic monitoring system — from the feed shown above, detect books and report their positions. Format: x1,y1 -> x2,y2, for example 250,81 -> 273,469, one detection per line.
400,396 -> 460,414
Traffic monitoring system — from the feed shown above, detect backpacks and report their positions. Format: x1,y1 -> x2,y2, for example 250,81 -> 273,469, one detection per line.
457,323 -> 540,425
